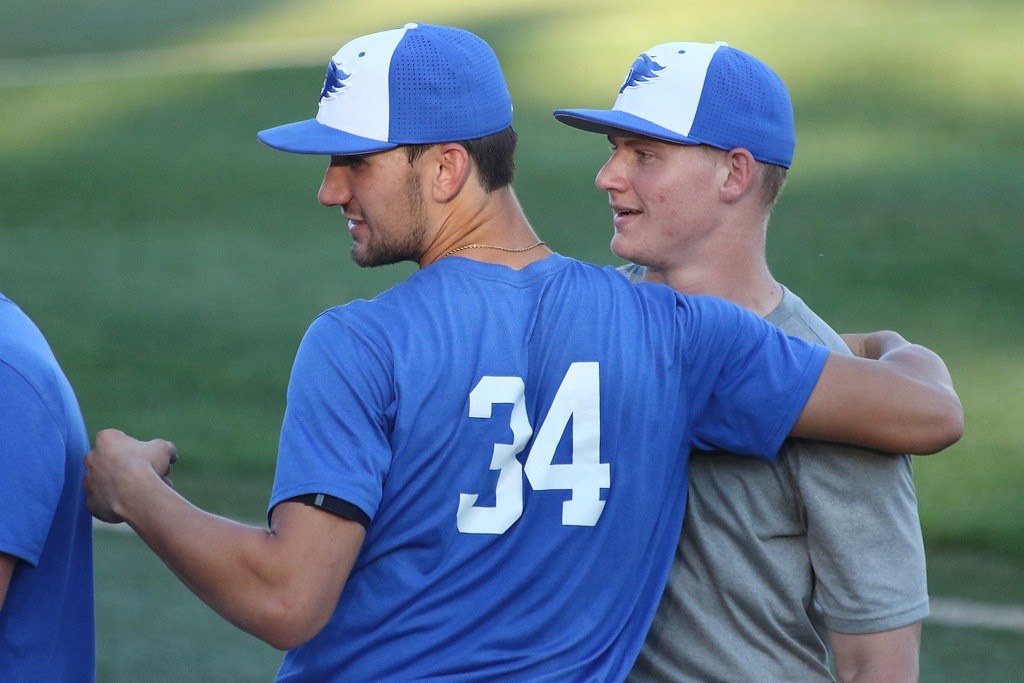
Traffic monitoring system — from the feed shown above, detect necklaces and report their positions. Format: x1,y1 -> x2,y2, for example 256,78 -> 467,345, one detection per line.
439,240 -> 546,256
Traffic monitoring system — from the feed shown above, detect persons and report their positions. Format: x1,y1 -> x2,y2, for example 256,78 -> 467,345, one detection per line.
82,23 -> 963,683
0,290 -> 96,683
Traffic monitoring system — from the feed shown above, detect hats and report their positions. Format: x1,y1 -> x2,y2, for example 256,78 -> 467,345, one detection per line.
258,20 -> 513,157
553,41 -> 796,169
